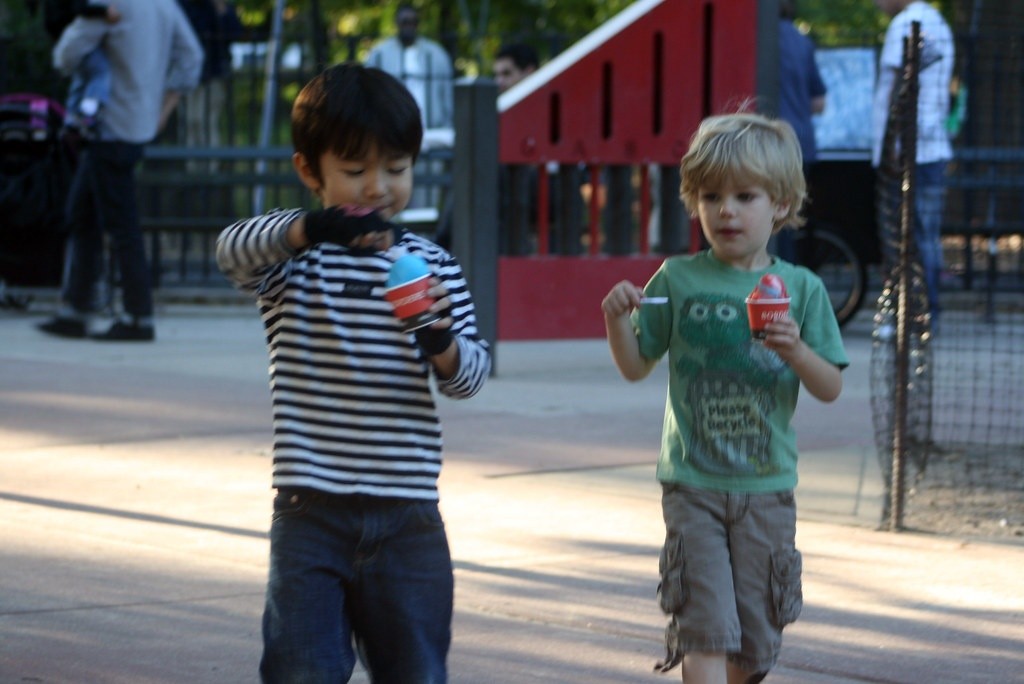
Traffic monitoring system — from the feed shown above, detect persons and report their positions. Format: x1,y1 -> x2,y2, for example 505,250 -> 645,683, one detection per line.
38,0 -> 956,343
214,61 -> 487,684
597,109 -> 849,684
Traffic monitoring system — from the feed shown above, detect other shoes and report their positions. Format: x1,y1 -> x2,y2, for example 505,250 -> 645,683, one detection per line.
39,316 -> 85,338
89,321 -> 153,340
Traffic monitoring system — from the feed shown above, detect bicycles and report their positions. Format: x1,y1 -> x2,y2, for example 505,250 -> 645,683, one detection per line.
780,221 -> 870,334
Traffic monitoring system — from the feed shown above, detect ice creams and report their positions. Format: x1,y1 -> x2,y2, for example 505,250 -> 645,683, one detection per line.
744,273 -> 791,343
383,253 -> 444,333
